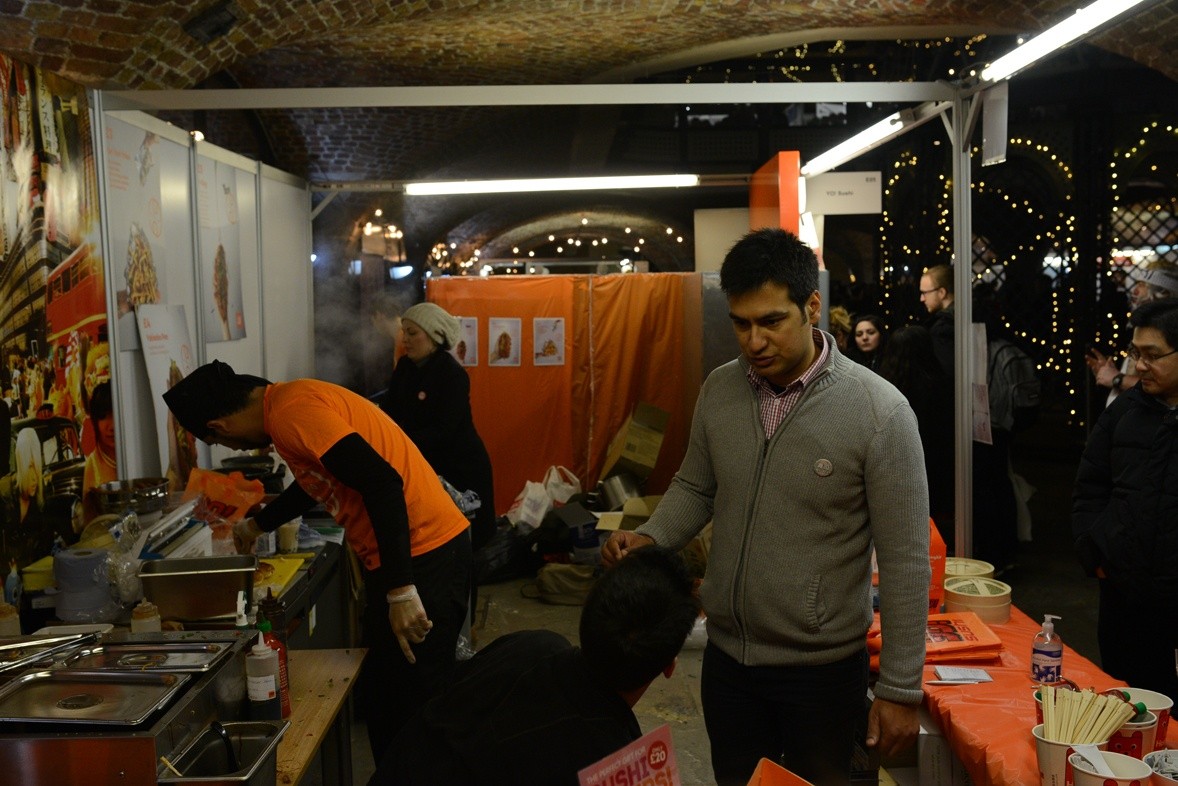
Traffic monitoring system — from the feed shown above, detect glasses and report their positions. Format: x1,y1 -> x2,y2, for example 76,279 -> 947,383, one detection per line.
920,288 -> 939,295
1126,349 -> 1178,364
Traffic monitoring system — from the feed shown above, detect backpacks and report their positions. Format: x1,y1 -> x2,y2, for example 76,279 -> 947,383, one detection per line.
988,336 -> 1042,436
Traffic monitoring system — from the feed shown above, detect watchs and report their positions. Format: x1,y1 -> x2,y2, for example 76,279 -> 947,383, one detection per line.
1111,374 -> 1127,391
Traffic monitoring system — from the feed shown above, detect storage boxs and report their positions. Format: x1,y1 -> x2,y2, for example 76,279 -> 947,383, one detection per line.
549,400 -> 711,577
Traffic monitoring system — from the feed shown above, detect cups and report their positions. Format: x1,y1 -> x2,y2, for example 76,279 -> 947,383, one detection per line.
278,514 -> 302,554
1033,681 -> 1178,786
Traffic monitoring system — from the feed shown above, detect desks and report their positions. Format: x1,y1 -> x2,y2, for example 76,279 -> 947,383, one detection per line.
859,581 -> 1117,786
229,647 -> 373,786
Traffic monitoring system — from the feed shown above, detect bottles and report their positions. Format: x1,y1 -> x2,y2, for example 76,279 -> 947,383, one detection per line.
1032,614 -> 1064,683
246,587 -> 292,722
131,596 -> 161,633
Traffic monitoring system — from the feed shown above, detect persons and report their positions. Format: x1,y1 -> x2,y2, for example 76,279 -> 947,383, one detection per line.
365,544 -> 700,785
0,321 -> 120,586
374,301 -> 495,665
368,296 -> 410,371
1087,266 -> 1177,408
161,359 -> 473,773
1070,295 -> 1178,721
828,256 -> 1039,579
594,228 -> 931,786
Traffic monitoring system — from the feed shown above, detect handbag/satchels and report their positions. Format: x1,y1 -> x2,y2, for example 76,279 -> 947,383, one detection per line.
541,465 -> 582,509
520,563 -> 593,605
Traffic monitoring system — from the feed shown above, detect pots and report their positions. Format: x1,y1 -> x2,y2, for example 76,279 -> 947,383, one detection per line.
87,477 -> 170,515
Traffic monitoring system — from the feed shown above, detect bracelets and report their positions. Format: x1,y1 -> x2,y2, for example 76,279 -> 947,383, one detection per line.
385,584 -> 417,604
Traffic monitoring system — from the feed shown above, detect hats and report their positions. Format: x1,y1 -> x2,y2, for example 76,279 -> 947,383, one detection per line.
161,359 -> 237,431
400,302 -> 459,351
1135,260 -> 1178,292
